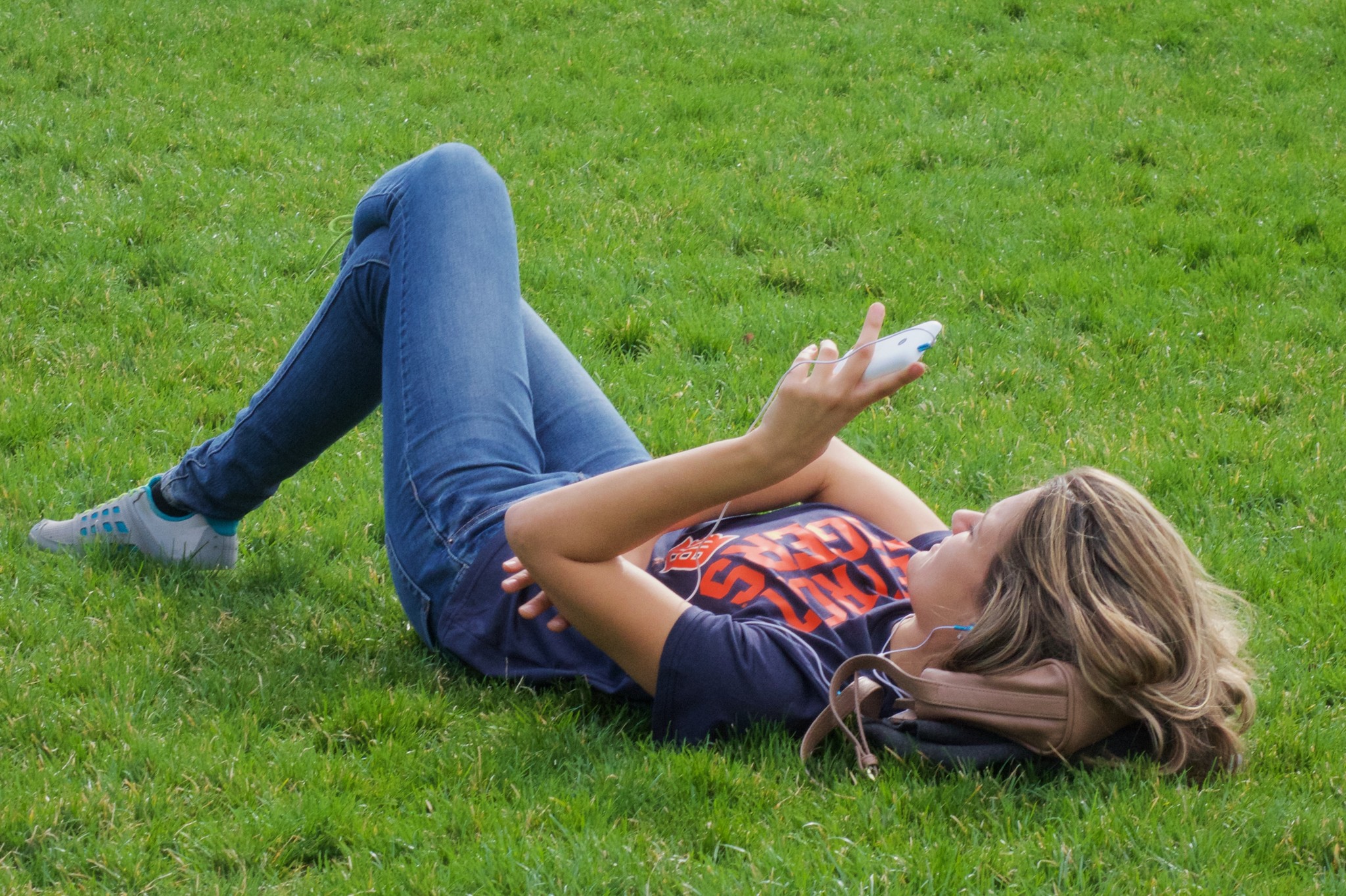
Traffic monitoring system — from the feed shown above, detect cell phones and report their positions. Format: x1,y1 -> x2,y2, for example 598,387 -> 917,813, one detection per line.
833,320 -> 944,384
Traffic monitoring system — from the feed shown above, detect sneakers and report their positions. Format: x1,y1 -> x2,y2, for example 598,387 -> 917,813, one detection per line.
27,474 -> 239,573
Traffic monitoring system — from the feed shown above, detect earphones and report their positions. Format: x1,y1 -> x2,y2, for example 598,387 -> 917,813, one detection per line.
954,624 -> 974,633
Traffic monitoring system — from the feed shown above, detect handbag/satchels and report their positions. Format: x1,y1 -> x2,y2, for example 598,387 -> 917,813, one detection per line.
798,655 -> 1129,800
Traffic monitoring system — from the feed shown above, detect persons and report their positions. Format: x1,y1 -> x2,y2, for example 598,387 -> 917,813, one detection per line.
29,142 -> 1261,785
